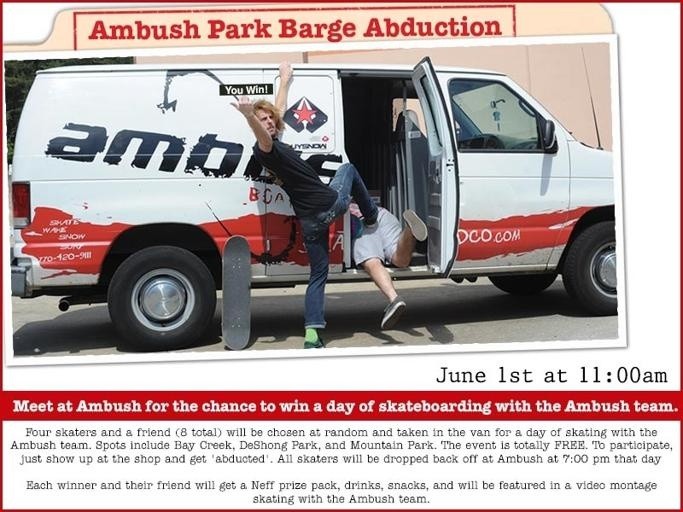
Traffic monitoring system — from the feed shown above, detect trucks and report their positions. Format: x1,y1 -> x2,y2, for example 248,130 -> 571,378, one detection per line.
11,57 -> 617,347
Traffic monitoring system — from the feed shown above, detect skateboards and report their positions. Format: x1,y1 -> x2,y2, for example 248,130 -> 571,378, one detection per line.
222,235 -> 251,349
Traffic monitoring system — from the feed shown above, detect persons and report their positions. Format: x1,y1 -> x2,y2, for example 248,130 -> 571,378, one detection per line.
227,59 -> 379,350
349,201 -> 428,331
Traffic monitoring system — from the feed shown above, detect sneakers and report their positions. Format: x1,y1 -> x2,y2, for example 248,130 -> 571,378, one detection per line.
381,299 -> 406,329
303,339 -> 325,349
366,223 -> 376,229
403,208 -> 428,242
